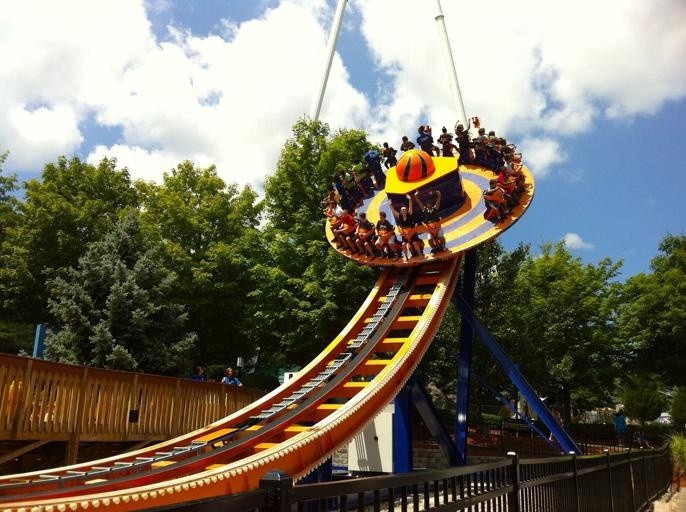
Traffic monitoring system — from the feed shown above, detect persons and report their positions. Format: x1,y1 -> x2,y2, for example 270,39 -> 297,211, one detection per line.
317,117 -> 532,262
190,365 -> 215,382
549,415 -> 565,439
614,409 -> 627,448
633,429 -> 655,451
220,367 -> 244,386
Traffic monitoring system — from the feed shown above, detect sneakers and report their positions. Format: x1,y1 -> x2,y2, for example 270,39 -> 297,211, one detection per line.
498,210 -> 513,223
379,248 -> 423,262
430,247 -> 447,257
331,238 -> 374,261
